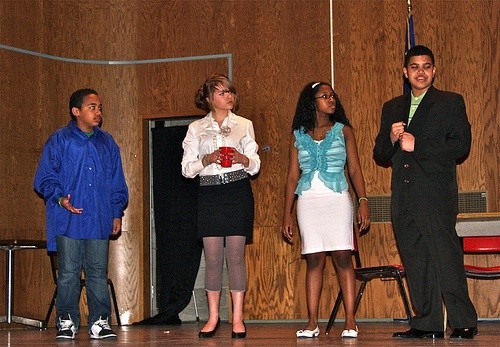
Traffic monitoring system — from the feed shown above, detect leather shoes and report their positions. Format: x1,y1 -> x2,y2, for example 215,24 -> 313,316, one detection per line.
392,327 -> 444,339
450,326 -> 480,340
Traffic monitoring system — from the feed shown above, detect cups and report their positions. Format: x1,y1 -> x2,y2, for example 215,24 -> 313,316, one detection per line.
218,147 -> 233,167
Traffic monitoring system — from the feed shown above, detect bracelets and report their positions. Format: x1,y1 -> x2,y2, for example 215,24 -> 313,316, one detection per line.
242,154 -> 246,166
202,154 -> 208,167
358,198 -> 368,203
58,196 -> 65,208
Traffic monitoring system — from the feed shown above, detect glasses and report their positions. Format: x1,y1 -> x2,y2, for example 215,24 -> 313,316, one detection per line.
315,94 -> 338,100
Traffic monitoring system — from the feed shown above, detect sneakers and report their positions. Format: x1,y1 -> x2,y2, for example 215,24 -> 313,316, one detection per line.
88,315 -> 117,341
56,314 -> 78,339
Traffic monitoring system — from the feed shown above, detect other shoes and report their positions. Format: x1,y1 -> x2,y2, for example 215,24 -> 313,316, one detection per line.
341,325 -> 359,338
296,325 -> 321,338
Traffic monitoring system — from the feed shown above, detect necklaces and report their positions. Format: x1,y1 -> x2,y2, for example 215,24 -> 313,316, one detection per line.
209,109 -> 231,137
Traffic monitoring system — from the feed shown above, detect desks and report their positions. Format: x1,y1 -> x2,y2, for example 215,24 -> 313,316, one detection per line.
0,240 -> 47,328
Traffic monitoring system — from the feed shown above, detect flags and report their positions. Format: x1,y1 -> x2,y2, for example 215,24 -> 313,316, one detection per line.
402,8 -> 416,97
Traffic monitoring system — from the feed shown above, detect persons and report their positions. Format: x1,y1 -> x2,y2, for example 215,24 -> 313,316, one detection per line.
282,81 -> 371,338
372,44 -> 478,340
180,72 -> 262,339
32,88 -> 130,341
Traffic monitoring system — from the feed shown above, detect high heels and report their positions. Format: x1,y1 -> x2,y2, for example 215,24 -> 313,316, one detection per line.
231,319 -> 247,340
199,317 -> 220,338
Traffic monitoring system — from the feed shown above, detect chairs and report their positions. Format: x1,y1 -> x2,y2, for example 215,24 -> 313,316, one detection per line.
44,251 -> 121,330
455,212 -> 500,322
325,219 -> 412,336
